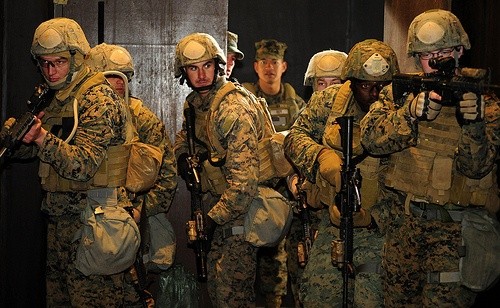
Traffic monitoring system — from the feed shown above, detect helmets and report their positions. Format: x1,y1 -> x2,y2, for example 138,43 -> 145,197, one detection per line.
30,17 -> 91,60
91,41 -> 134,83
303,49 -> 349,86
405,9 -> 471,58
175,32 -> 226,78
340,39 -> 400,81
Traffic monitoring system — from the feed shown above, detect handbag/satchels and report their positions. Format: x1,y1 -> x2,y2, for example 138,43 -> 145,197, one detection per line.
242,184 -> 293,247
448,210 -> 500,291
74,197 -> 141,275
147,213 -> 178,272
122,141 -> 163,192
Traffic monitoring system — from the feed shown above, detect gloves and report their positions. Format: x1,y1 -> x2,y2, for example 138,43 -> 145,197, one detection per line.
289,176 -> 300,200
328,205 -> 372,228
458,92 -> 485,121
317,148 -> 345,193
186,216 -> 216,250
177,152 -> 194,182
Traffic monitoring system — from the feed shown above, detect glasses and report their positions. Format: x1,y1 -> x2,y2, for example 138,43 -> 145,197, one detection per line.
418,44 -> 464,60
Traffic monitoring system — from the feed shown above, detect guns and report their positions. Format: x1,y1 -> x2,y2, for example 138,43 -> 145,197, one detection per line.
180,108 -> 211,285
334,115 -> 362,308
295,178 -> 317,268
0,82 -> 54,164
391,56 -> 500,121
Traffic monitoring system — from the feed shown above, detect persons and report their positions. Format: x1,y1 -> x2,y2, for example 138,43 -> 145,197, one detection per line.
4,8 -> 500,308
241,40 -> 307,132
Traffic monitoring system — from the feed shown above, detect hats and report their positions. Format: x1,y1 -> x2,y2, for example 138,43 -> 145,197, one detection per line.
254,38 -> 287,60
227,31 -> 245,60
